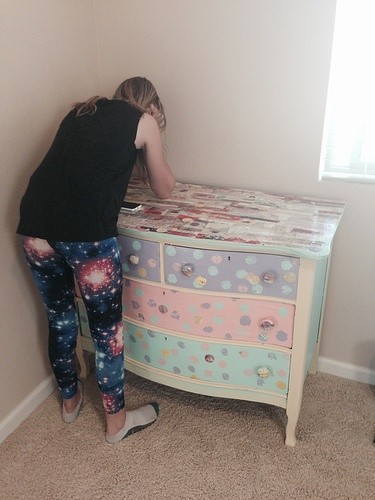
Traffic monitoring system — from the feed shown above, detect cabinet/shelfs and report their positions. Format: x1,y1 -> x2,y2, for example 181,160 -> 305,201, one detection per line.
72,175 -> 345,447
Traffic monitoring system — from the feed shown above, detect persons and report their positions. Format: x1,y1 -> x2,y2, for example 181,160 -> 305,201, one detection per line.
18,75 -> 180,444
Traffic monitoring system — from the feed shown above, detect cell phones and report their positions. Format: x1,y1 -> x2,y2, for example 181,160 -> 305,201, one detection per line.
120,201 -> 142,212
